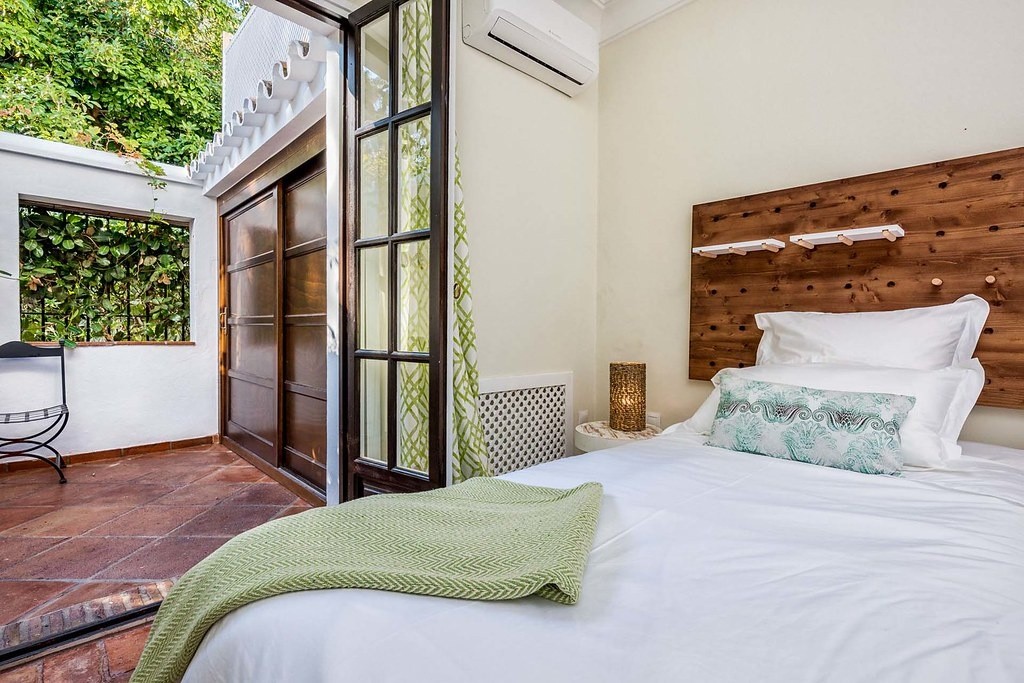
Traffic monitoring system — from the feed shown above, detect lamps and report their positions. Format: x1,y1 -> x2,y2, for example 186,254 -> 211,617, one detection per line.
610,361 -> 647,431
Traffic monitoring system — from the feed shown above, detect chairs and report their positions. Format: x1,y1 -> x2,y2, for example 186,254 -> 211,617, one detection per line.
0,339 -> 70,484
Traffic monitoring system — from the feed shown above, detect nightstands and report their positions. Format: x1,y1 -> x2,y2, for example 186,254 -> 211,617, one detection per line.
575,420 -> 663,453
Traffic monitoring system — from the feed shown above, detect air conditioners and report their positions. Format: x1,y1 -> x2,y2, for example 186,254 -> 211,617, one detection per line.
462,0 -> 600,99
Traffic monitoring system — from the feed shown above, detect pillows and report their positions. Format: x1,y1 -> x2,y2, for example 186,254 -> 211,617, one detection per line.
702,373 -> 917,476
664,358 -> 986,472
755,293 -> 991,367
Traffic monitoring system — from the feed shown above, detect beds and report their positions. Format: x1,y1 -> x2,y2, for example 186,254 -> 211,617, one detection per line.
126,420 -> 1024,683
